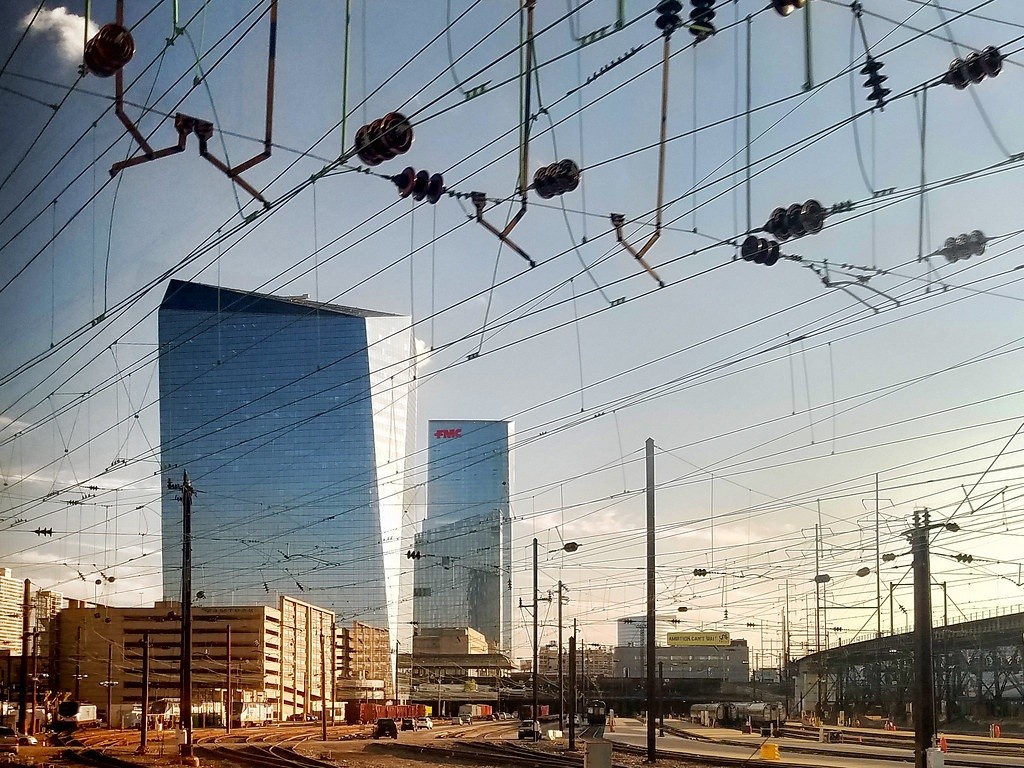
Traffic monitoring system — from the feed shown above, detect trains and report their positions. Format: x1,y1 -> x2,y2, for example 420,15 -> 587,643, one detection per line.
690,701 -> 787,729
587,700 -> 606,725
520,705 -> 549,721
343,703 -> 432,726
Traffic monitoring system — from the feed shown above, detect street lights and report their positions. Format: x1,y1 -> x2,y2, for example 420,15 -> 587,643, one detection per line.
214,688 -> 227,726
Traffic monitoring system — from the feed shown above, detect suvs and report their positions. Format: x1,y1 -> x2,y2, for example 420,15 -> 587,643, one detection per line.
451,714 -> 473,727
370,718 -> 399,740
565,714 -> 581,728
486,711 -> 518,722
400,717 -> 433,732
516,721 -> 543,739
0,726 -> 38,755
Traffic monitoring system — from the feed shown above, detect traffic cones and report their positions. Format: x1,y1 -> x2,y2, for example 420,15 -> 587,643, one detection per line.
939,738 -> 947,754
